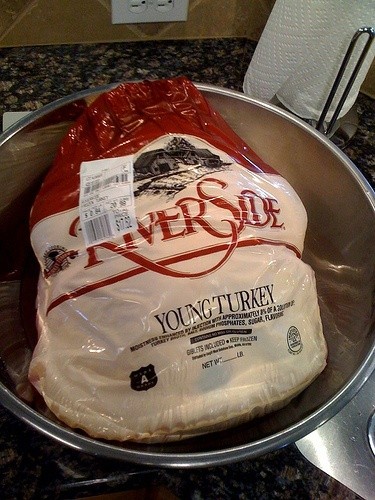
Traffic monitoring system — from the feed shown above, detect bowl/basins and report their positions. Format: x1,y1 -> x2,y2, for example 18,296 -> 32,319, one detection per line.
0,78 -> 375,468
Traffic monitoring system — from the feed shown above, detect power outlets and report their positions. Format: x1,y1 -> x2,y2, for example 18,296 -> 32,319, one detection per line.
110,0 -> 188,25
